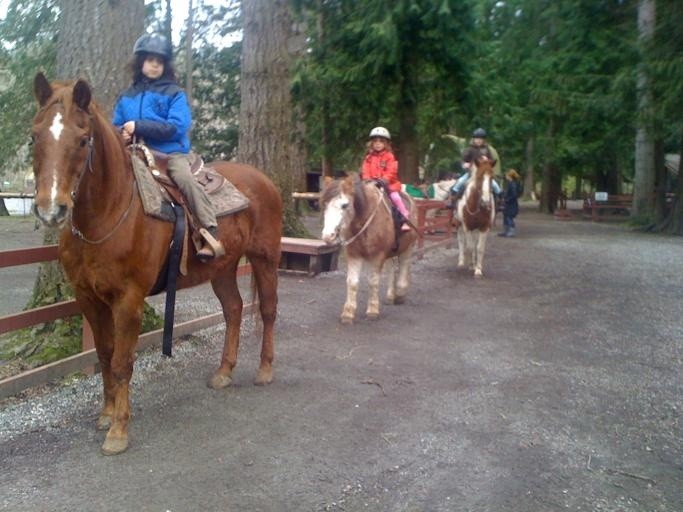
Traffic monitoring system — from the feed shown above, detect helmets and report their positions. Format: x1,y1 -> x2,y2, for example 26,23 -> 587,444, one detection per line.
369,126 -> 391,140
132,31 -> 169,57
473,128 -> 485,138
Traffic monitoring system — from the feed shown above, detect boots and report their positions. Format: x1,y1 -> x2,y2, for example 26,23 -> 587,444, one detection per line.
498,224 -> 516,236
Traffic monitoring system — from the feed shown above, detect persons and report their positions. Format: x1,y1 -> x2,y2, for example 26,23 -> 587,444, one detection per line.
419,169 -> 462,187
442,126 -> 504,211
496,169 -> 523,238
107,30 -> 223,261
361,125 -> 411,232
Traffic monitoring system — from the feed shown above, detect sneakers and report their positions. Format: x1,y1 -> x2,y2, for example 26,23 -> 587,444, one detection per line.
198,240 -> 225,256
401,213 -> 413,231
447,199 -> 454,208
497,206 -> 505,210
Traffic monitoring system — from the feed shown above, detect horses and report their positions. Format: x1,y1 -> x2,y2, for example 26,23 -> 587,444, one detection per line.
401,178 -> 456,236
452,157 -> 499,278
30,71 -> 284,457
318,175 -> 419,327
